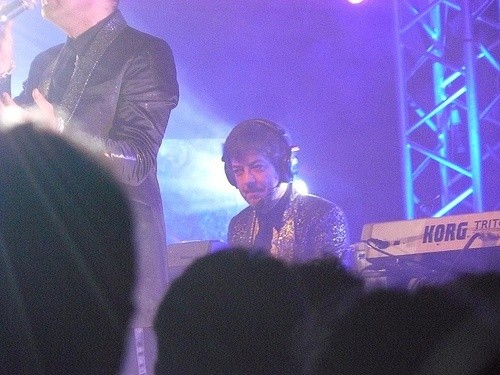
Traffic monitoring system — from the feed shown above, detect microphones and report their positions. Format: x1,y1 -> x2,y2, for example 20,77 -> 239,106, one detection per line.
370,238 -> 389,249
479,228 -> 500,238
0,0 -> 36,26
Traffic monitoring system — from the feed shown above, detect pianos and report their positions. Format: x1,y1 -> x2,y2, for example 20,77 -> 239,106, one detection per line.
359,211 -> 500,261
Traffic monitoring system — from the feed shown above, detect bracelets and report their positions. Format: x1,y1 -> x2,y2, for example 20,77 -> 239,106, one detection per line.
0,60 -> 14,79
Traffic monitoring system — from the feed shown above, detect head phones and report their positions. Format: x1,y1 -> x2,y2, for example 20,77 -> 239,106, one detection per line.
224,119 -> 298,186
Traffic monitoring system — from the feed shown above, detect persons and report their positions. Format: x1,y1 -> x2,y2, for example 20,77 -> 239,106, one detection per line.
222,118 -> 348,265
0,0 -> 179,374
0,124 -> 500,375
